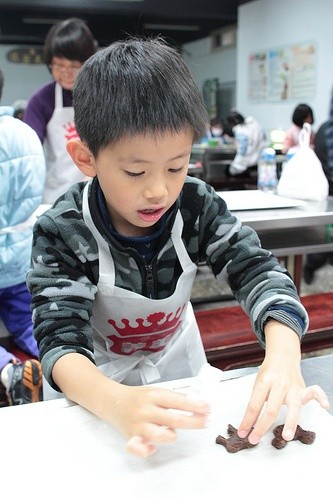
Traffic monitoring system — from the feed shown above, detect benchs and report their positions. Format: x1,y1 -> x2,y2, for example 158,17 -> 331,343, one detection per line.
204,174 -> 258,190
0,293 -> 333,406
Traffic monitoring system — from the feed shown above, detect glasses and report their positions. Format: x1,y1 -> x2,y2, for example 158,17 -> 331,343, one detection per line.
48,63 -> 82,74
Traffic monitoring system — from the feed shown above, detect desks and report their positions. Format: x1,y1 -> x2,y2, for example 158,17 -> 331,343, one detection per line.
0,143 -> 333,500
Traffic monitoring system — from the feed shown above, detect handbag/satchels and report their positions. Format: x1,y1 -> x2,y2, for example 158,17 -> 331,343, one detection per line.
277,122 -> 329,202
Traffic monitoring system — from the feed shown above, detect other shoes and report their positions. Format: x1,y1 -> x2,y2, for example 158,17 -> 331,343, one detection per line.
6,358 -> 42,407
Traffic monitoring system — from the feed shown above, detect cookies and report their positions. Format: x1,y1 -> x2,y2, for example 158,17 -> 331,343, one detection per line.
215,424 -> 259,453
271,424 -> 315,449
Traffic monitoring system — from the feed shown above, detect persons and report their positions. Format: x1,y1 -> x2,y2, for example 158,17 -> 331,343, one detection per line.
25,37 -> 330,456
0,17 -> 98,407
199,104 -> 333,193
